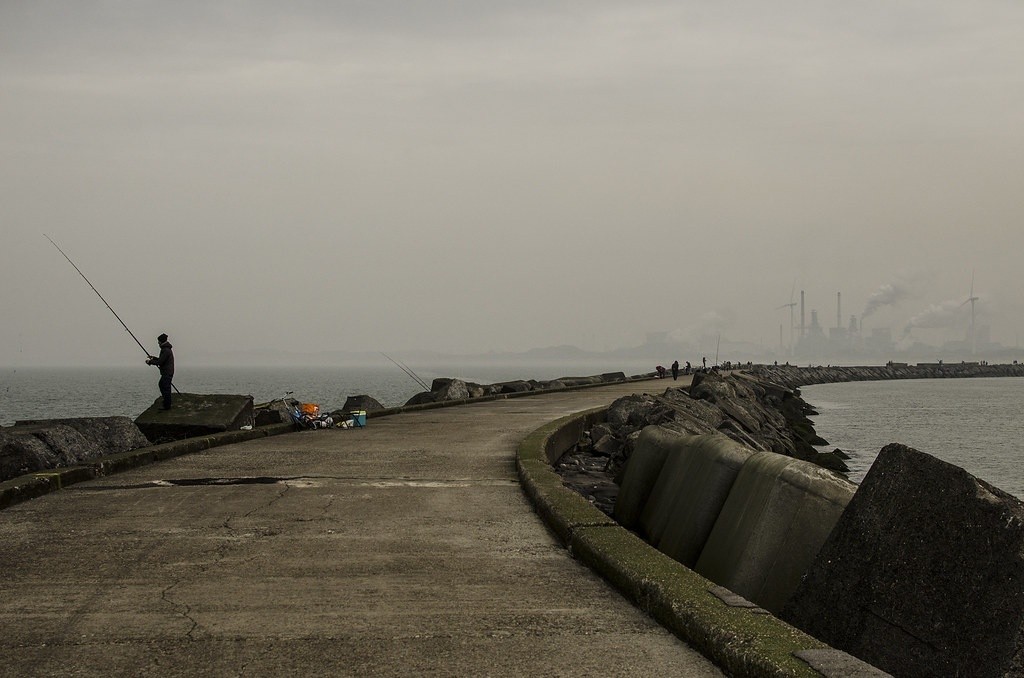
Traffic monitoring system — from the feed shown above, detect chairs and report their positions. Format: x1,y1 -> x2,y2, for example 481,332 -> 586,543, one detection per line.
333,396 -> 364,430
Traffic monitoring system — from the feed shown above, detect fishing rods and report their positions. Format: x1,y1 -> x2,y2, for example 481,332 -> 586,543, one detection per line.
40,231 -> 186,401
374,346 -> 432,393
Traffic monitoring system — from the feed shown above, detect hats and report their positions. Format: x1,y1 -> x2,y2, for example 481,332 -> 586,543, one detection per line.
157,333 -> 168,343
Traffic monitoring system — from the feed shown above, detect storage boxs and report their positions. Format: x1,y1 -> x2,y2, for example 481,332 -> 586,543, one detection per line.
350,410 -> 366,426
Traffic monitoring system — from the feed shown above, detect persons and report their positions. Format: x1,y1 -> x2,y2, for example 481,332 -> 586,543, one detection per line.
672,361 -> 678,380
703,357 -> 753,371
774,360 -> 788,366
145,333 -> 175,410
656,366 -> 665,379
686,361 -> 691,375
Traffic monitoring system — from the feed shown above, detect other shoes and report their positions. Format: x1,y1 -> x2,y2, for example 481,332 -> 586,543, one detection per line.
158,407 -> 165,410
161,398 -> 164,402
